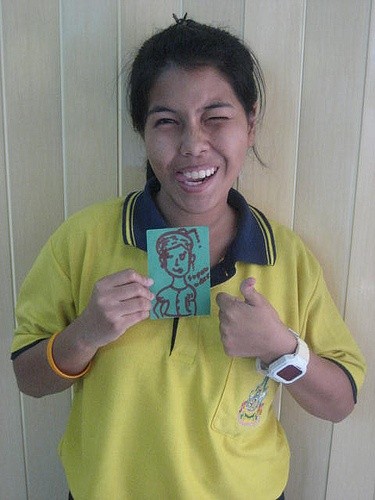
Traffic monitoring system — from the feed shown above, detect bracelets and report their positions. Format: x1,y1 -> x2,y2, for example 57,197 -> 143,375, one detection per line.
46,332 -> 92,381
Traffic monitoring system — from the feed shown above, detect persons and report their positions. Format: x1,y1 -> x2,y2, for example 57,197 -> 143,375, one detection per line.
11,19 -> 366,500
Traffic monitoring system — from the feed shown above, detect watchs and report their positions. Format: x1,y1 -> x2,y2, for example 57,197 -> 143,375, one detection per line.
255,328 -> 310,383
